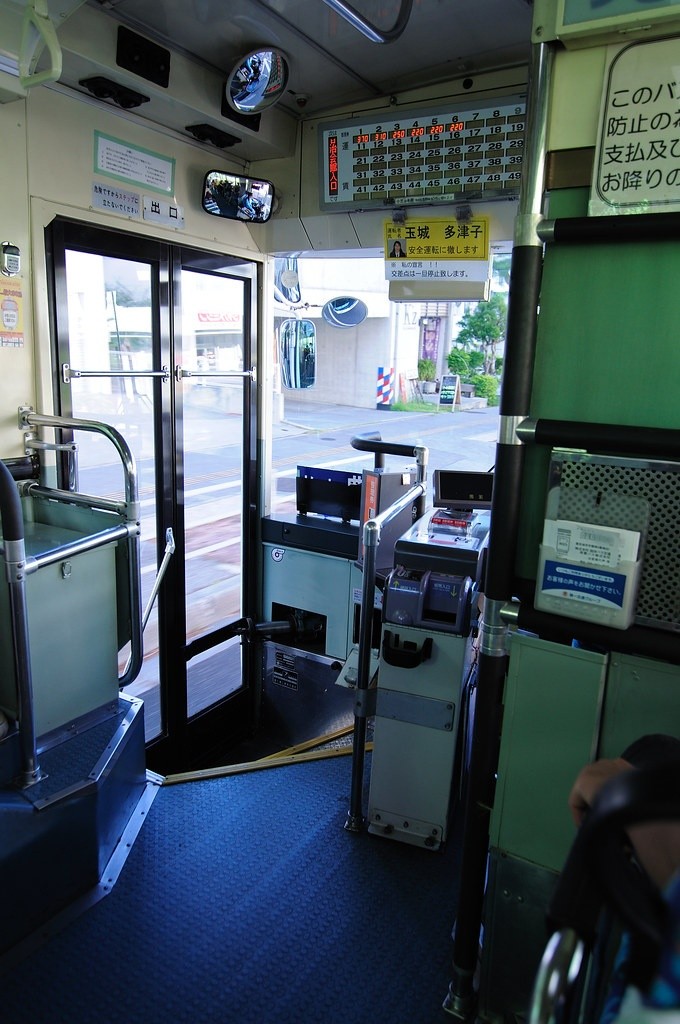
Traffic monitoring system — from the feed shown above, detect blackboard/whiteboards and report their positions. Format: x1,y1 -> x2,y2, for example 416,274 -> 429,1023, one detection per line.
438,375 -> 462,406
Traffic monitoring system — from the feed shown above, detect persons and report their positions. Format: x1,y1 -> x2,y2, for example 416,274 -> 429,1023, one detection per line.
572,731 -> 680,1024
250,53 -> 263,82
304,342 -> 313,359
210,180 -> 256,220
